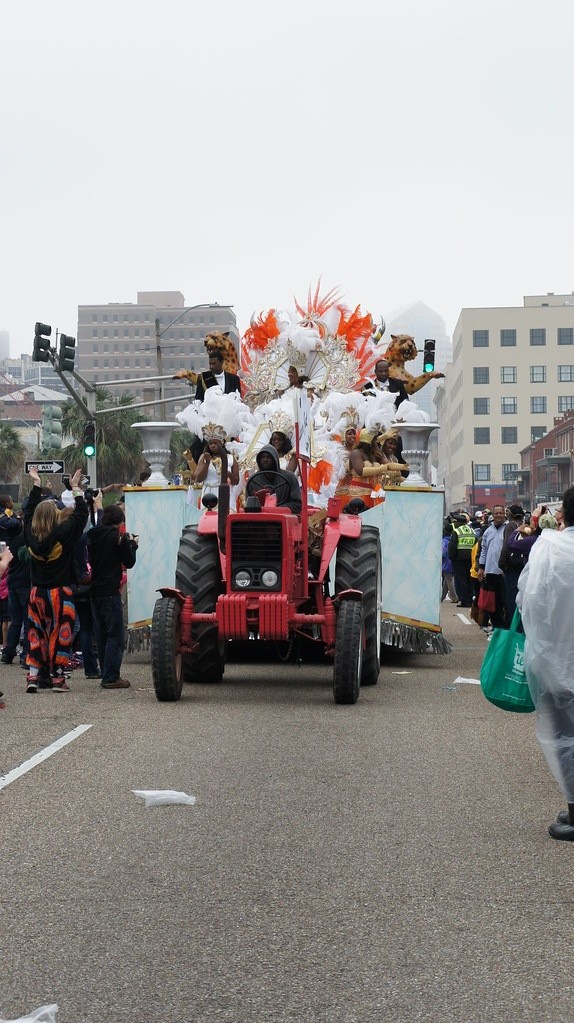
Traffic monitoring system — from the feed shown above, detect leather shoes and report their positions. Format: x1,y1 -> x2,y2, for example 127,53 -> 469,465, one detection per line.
557,810 -> 574,825
548,822 -> 574,841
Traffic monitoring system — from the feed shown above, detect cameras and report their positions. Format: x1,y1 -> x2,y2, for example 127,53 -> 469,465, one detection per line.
85,488 -> 102,503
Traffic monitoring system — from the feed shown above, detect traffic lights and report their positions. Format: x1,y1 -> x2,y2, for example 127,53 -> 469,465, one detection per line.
83,423 -> 95,457
33,321 -> 52,362
58,333 -> 76,372
423,339 -> 436,374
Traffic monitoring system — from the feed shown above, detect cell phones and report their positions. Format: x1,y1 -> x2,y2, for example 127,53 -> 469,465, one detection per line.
541,506 -> 546,515
525,512 -> 531,526
0,541 -> 6,554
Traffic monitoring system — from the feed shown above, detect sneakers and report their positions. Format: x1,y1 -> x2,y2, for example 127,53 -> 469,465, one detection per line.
25,677 -> 38,693
52,678 -> 70,691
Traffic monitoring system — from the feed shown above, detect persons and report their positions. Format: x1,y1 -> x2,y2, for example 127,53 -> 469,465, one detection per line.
362,360 -> 408,410
1,480 -> 126,696
134,472 -> 149,486
194,352 -> 241,403
184,422 -> 240,515
248,445 -> 302,514
268,409 -> 297,478
275,366 -> 304,400
23,466 -> 88,691
442,503 -> 565,630
520,487 -> 574,841
87,504 -> 140,688
337,425 -> 410,516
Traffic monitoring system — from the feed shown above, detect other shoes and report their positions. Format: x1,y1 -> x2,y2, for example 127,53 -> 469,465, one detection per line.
63,671 -> 71,679
86,668 -> 103,679
457,604 -> 471,607
100,679 -> 130,688
21,659 -> 29,670
1,656 -> 13,664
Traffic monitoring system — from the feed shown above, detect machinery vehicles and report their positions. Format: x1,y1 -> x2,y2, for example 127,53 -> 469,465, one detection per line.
150,468 -> 383,705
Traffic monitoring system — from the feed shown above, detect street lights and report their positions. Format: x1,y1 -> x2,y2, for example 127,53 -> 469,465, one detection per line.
155,300 -> 234,423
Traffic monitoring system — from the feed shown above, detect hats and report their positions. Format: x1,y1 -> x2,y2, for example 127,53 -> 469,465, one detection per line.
453,514 -> 466,521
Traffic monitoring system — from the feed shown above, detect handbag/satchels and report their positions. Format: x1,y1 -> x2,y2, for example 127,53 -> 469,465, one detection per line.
479,608 -> 540,713
470,579 -> 496,624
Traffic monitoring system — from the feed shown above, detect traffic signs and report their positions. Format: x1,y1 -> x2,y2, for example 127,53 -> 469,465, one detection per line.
24,460 -> 66,475
62,475 -> 91,486
